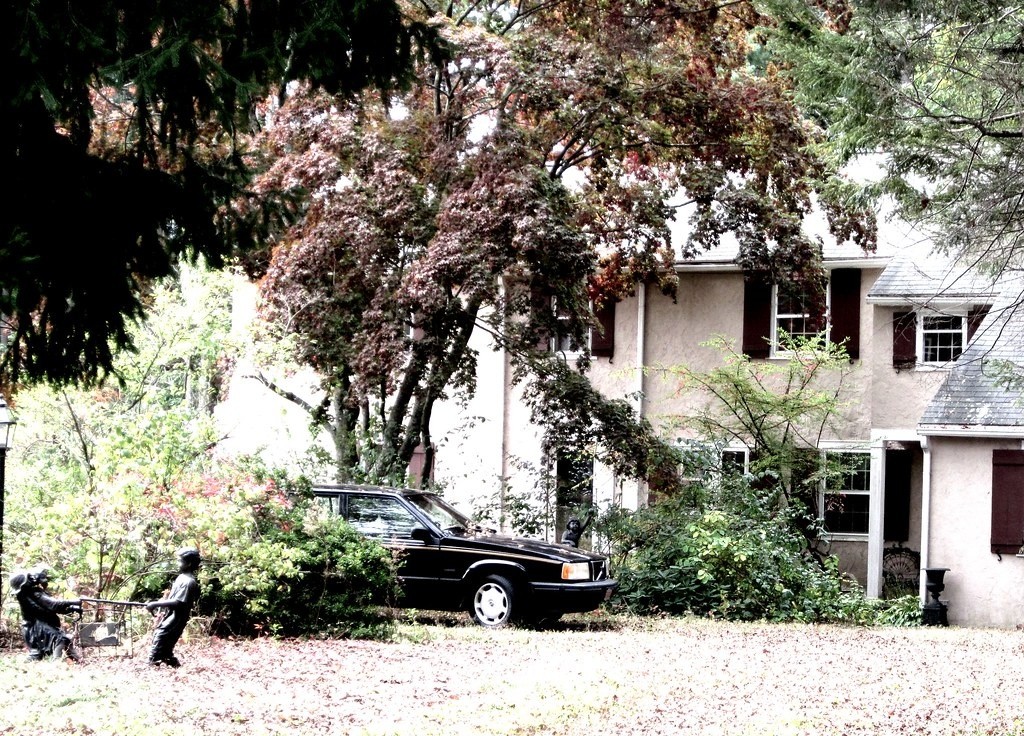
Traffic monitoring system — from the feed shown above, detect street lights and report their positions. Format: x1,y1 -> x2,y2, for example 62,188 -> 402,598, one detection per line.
0,394 -> 18,615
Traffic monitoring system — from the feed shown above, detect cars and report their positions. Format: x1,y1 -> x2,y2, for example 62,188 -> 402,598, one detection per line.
301,484 -> 620,630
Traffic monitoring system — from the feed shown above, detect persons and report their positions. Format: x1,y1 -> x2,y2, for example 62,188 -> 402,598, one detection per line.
9,566 -> 83,665
561,508 -> 594,548
146,548 -> 201,669
